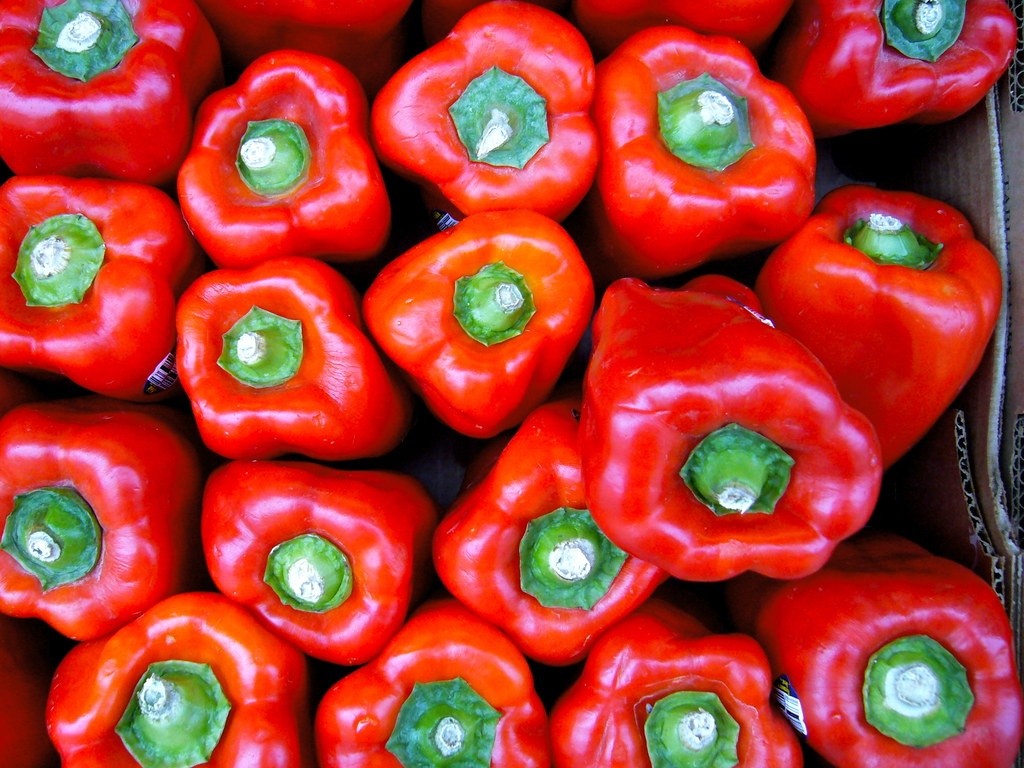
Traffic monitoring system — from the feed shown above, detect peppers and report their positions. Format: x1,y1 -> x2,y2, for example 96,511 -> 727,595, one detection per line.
0,0 -> 1024,768
